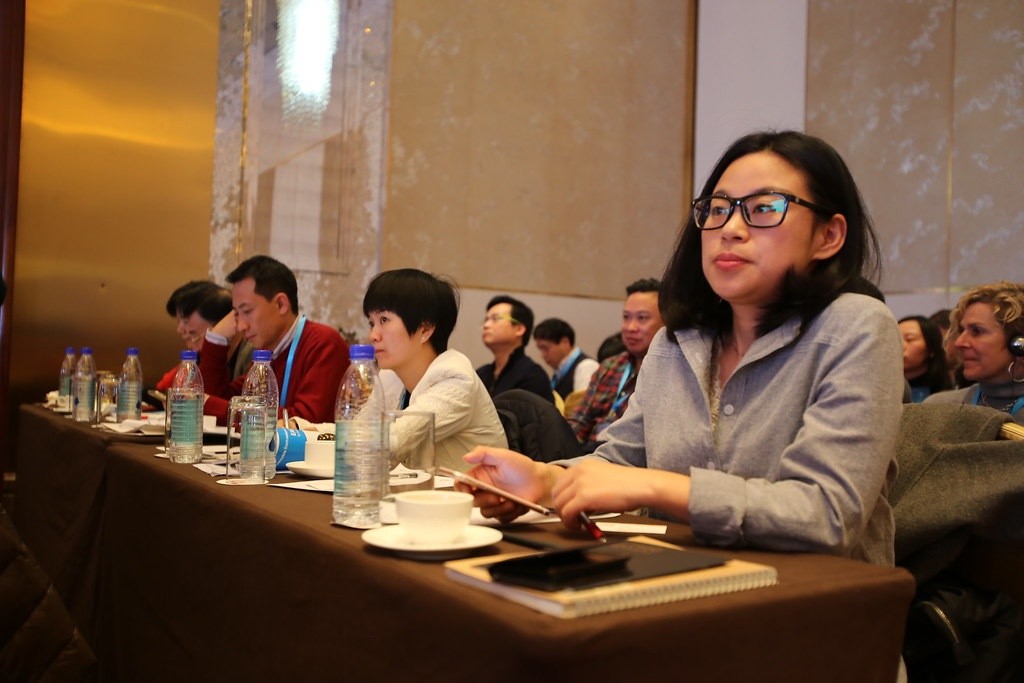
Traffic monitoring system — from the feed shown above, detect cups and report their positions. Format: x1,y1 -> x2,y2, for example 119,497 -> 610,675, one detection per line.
165,388 -> 175,455
382,410 -> 436,490
68,370 -> 117,422
226,395 -> 266,484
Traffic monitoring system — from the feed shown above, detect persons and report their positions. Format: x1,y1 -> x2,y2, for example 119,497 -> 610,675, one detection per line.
566,277 -> 662,457
171,255 -> 351,433
898,314 -> 954,404
475,294 -> 556,407
276,267 -> 510,473
533,318 -> 600,420
927,309 -> 971,391
455,130 -> 908,683
597,331 -> 627,365
142,280 -> 248,413
920,280 -> 1024,423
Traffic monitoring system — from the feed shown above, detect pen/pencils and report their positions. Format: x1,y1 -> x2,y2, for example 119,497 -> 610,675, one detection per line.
283,409 -> 289,429
389,473 -> 418,479
580,511 -> 607,543
215,451 -> 240,454
141,416 -> 148,419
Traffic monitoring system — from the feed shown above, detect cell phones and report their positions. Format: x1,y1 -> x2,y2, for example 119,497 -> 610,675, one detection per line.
439,467 -> 550,517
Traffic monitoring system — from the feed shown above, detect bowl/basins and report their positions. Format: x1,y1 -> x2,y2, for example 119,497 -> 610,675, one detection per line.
396,489 -> 474,546
304,441 -> 335,466
204,415 -> 216,432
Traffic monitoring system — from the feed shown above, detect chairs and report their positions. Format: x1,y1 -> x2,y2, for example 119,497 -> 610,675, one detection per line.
493,389 -> 580,465
880,388 -> 1024,683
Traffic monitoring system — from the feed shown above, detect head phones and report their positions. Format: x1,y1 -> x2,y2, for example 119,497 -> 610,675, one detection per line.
1008,334 -> 1024,357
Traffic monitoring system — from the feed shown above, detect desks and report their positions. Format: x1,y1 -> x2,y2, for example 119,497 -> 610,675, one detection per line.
0,401 -> 916,683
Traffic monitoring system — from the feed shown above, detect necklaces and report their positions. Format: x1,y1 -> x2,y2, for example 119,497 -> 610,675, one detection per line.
979,389 -> 1020,414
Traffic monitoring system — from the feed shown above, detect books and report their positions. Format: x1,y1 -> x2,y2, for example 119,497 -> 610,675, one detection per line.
443,533 -> 779,621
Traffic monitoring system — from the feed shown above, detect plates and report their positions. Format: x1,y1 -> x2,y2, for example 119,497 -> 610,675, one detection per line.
362,526 -> 504,559
285,461 -> 334,478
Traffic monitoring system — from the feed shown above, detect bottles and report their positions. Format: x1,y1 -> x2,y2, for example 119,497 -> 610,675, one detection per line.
59,347 -> 76,396
117,347 -> 143,422
241,349 -> 279,479
73,347 -> 96,422
333,345 -> 384,528
170,349 -> 204,465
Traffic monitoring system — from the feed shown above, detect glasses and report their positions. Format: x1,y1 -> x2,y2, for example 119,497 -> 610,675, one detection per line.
480,314 -> 523,327
692,187 -> 842,231
